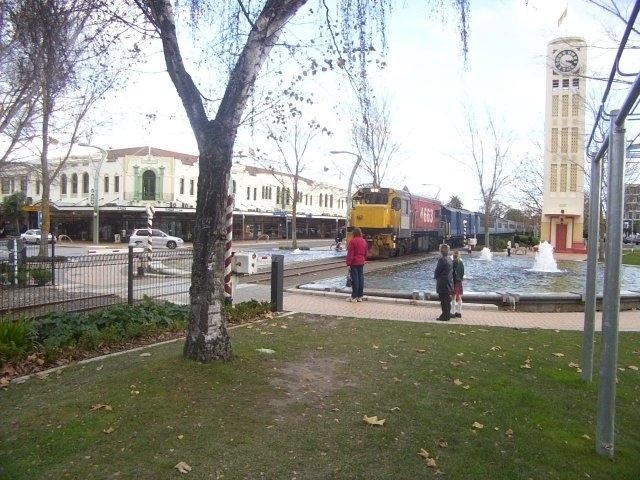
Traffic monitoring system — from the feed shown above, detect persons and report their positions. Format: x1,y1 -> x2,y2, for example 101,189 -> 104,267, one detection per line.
433,243 -> 454,320
506,238 -> 511,257
514,232 -> 521,251
344,227 -> 369,303
452,248 -> 466,318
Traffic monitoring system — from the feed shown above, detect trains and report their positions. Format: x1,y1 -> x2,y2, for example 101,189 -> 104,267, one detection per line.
346,186 -> 533,260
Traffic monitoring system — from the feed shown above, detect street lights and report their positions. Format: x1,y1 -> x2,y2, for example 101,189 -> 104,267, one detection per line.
78,142 -> 108,244
331,150 -> 362,229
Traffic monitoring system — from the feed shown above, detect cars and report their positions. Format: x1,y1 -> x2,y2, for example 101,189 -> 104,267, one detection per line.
21,228 -> 57,245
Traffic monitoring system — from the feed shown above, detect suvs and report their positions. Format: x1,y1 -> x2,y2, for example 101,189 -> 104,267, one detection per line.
130,227 -> 186,249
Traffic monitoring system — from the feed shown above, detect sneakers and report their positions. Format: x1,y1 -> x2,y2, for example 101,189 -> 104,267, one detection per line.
346,298 -> 362,303
437,313 -> 461,320
